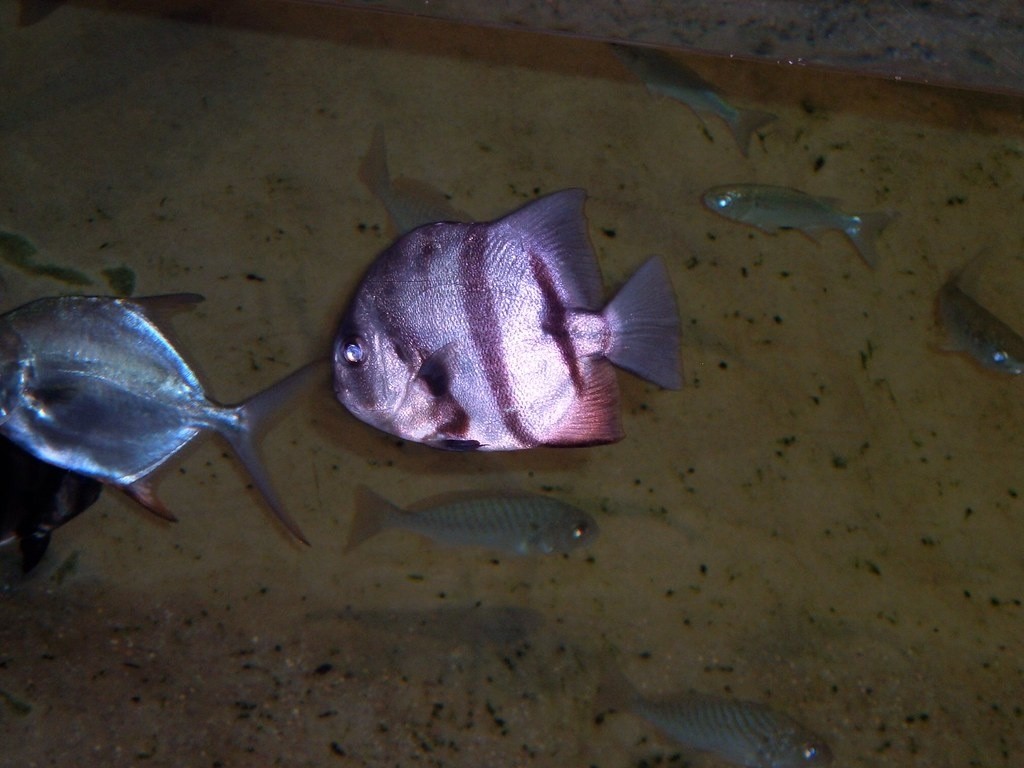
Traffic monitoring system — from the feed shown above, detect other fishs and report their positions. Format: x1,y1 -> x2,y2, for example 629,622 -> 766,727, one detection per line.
0,39 -> 1024,768
